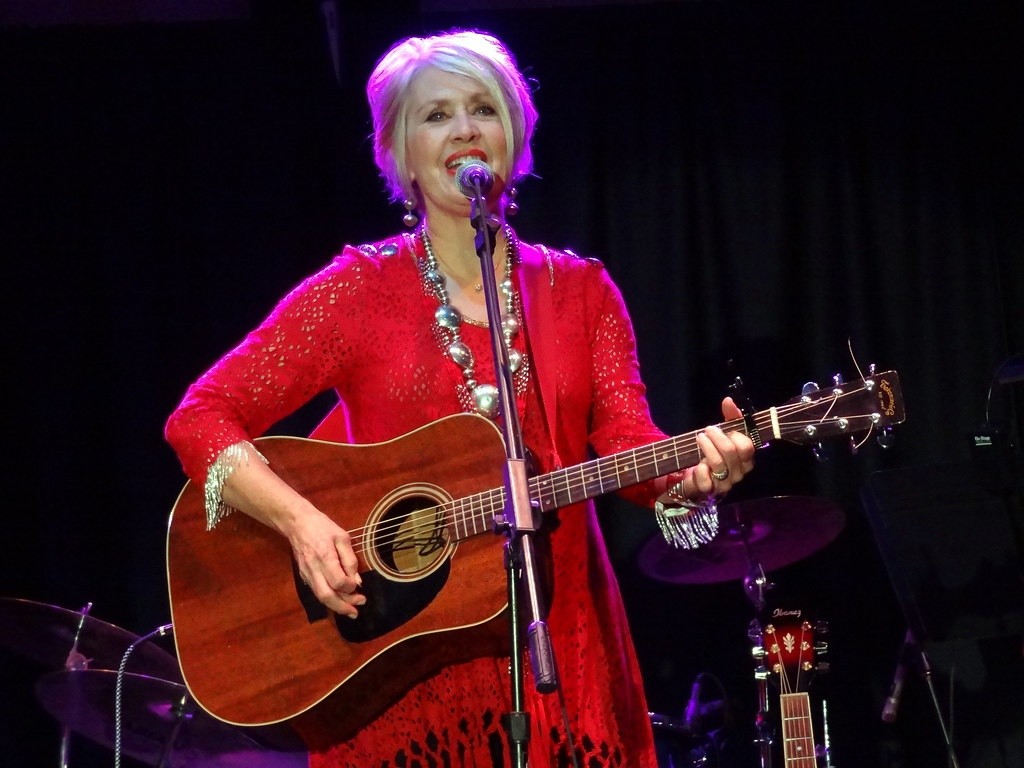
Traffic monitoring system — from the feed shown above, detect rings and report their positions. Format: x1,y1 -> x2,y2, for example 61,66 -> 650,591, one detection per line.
713,471 -> 728,480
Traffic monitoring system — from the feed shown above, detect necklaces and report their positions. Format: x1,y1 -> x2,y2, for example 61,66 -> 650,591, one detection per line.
419,219 -> 522,418
432,242 -> 507,291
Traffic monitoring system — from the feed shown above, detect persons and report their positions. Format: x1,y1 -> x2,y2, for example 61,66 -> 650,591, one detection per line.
682,672 -> 737,768
159,27 -> 753,768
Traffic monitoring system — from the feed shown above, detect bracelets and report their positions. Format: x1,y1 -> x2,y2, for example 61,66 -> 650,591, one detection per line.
667,482 -> 710,509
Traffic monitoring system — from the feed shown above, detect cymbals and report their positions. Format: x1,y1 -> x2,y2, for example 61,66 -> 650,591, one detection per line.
35,668 -> 310,768
637,494 -> 843,584
0,595 -> 186,687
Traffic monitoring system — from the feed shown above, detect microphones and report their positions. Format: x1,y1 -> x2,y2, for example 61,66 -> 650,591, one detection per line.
885,627 -> 917,723
457,159 -> 496,198
685,675 -> 706,731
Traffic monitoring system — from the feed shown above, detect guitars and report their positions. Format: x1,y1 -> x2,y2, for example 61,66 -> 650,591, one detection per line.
746,606 -> 833,768
165,361 -> 909,729
